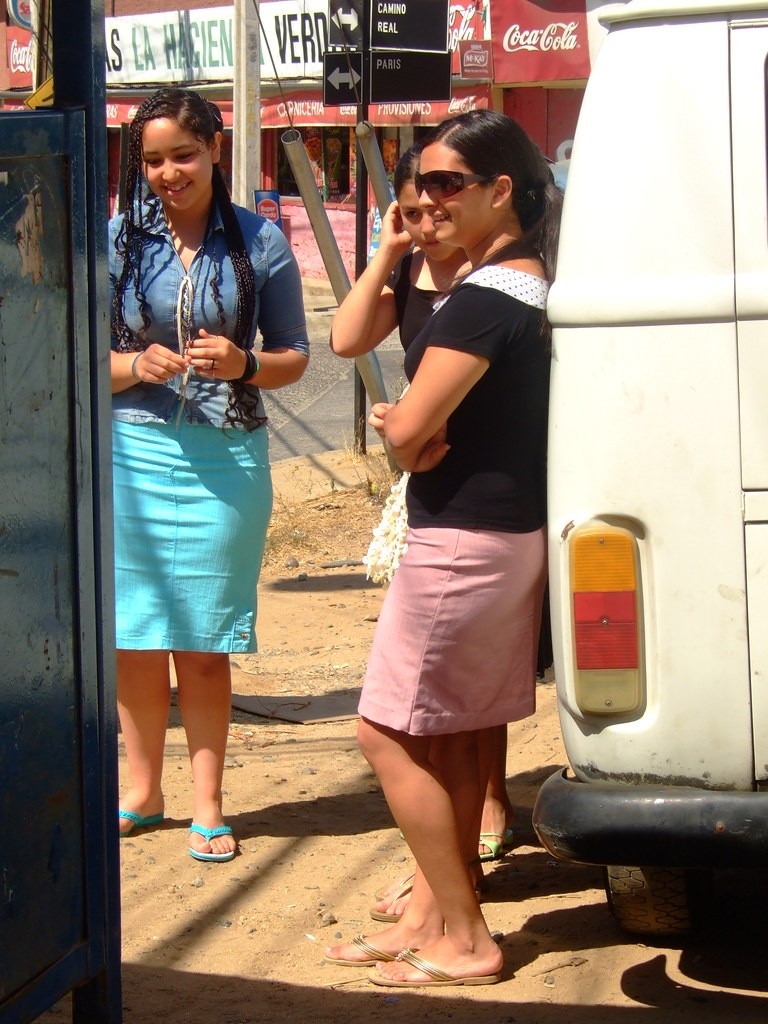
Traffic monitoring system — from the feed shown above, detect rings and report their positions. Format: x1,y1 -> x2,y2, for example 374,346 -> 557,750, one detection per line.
209,359 -> 214,370
215,335 -> 218,339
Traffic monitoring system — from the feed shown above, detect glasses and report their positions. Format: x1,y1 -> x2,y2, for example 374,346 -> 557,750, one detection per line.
414,170 -> 489,198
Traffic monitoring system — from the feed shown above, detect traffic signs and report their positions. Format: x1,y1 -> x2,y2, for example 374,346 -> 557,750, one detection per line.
324,0 -> 454,107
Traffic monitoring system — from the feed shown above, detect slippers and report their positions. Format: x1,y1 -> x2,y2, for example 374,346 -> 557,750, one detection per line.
119,809 -> 165,837
324,934 -> 397,968
375,874 -> 418,902
187,823 -> 235,862
368,947 -> 500,986
478,830 -> 513,860
371,879 -> 481,922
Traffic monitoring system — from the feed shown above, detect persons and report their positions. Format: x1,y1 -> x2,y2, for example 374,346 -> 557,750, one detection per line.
109,89 -> 308,863
323,108 -> 565,987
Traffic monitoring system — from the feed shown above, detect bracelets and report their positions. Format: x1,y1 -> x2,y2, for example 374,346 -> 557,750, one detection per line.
131,351 -> 144,380
238,347 -> 260,383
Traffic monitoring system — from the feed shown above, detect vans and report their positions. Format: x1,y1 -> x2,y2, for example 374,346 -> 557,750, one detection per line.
533,0 -> 768,945
